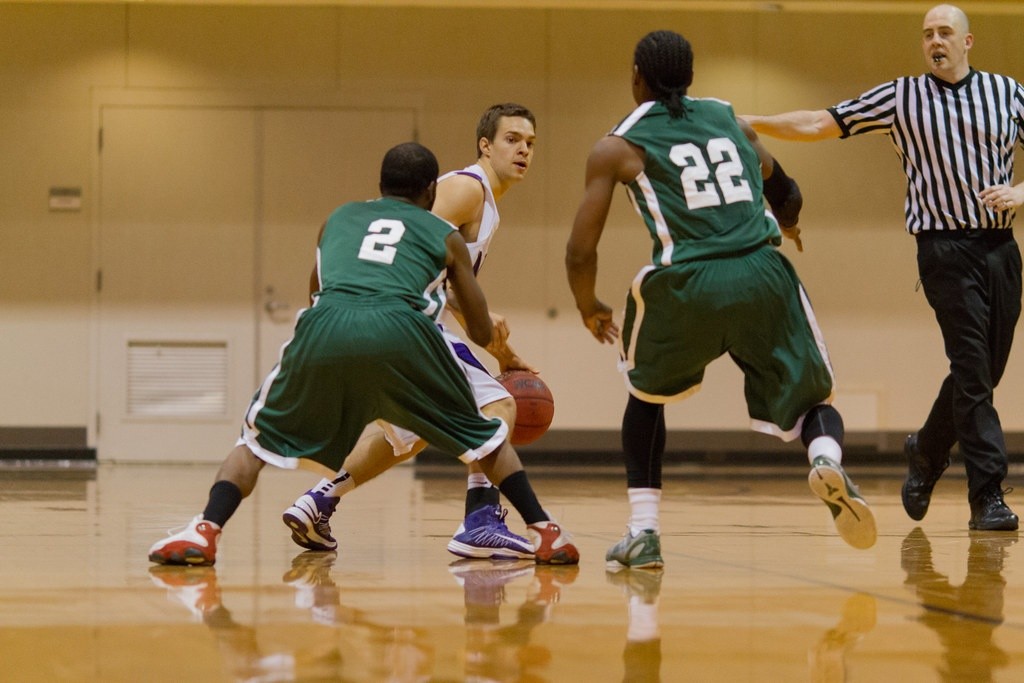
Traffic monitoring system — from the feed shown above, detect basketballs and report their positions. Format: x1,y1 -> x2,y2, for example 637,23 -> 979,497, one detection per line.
493,368 -> 556,447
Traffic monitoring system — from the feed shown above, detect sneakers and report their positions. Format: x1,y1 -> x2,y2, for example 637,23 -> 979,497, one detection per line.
605,524 -> 666,568
147,513 -> 223,567
808,457 -> 875,549
445,504 -> 534,559
526,521 -> 579,564
901,434 -> 950,522
282,490 -> 339,550
968,487 -> 1018,529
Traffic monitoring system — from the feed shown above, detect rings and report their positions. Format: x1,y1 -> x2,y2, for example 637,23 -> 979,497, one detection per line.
1002,201 -> 1008,208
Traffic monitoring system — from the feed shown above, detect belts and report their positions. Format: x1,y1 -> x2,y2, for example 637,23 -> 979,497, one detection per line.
918,228 -> 1013,241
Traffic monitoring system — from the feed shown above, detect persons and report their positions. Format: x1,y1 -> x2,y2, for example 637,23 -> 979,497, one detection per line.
146,141 -> 580,566
281,102 -> 541,562
565,29 -> 878,575
734,5 -> 1024,530
